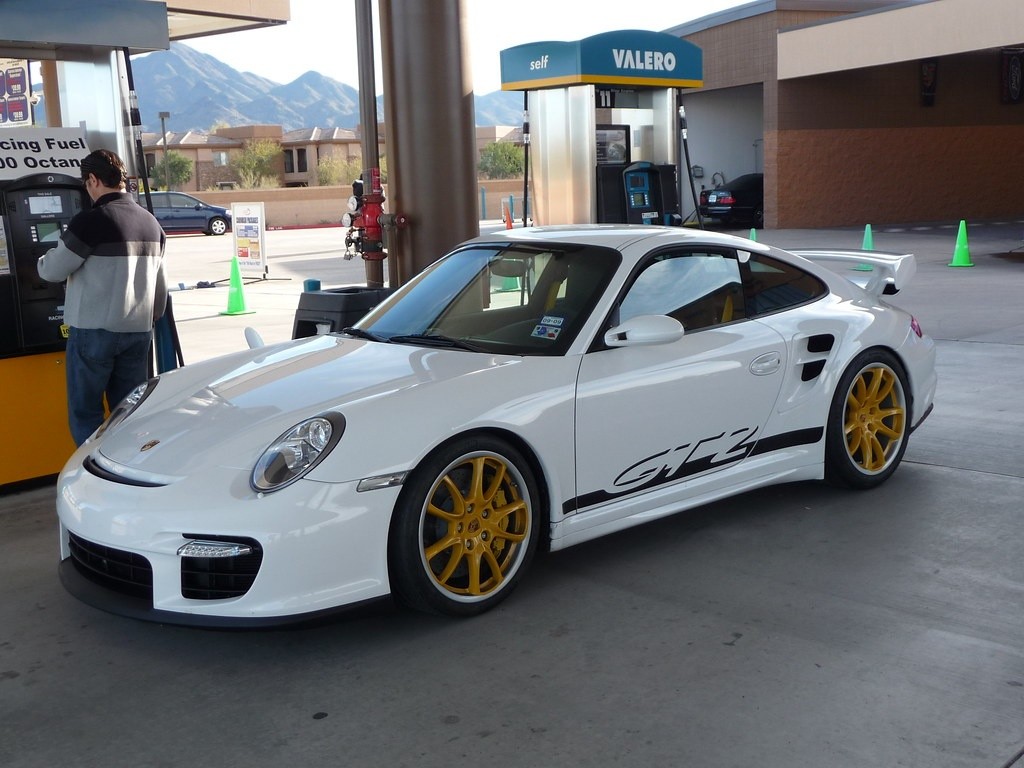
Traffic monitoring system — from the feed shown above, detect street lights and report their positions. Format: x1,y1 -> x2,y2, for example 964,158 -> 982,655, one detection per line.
159,110 -> 170,207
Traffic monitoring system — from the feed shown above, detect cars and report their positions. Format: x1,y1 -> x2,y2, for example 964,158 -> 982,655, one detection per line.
54,222 -> 939,632
137,192 -> 232,236
698,173 -> 764,228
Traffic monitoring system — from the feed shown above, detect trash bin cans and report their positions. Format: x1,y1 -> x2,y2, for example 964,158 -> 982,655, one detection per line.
291,285 -> 395,340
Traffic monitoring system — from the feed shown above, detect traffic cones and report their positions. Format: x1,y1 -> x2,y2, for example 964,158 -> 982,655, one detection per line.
749,227 -> 757,242
495,256 -> 522,292
851,222 -> 874,272
948,220 -> 975,267
220,256 -> 255,315
506,209 -> 513,230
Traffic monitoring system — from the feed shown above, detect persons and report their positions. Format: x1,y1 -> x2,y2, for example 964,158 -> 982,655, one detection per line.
36,150 -> 167,450
